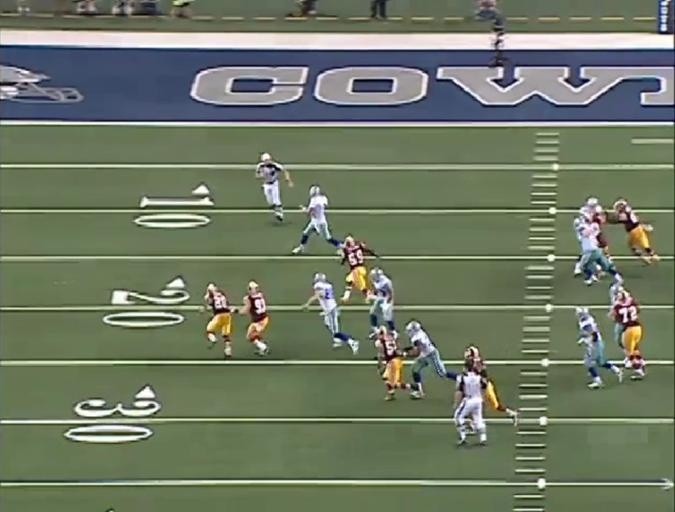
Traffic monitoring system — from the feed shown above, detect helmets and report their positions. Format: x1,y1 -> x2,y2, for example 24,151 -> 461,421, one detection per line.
310,183 -> 319,195
246,280 -> 258,292
207,282 -> 217,289
314,271 -> 326,281
404,320 -> 420,333
613,199 -> 626,210
260,151 -> 270,162
463,345 -> 478,361
576,305 -> 587,316
573,219 -> 584,231
369,267 -> 381,279
609,280 -> 623,293
586,197 -> 598,205
345,235 -> 353,246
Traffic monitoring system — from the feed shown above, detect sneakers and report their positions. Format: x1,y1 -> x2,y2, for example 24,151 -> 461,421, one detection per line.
273,211 -> 283,220
587,359 -> 644,388
340,296 -> 349,301
410,391 -> 425,399
642,256 -> 660,267
458,426 -> 487,446
572,265 -> 625,287
291,245 -> 304,255
367,331 -> 378,340
224,350 -> 231,359
513,411 -> 519,427
331,339 -> 359,355
252,346 -> 271,359
384,392 -> 396,400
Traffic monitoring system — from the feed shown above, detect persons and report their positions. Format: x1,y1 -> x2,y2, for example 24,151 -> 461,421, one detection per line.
297,0 -> 318,17
204,283 -> 233,358
574,196 -> 660,390
73,1 -> 191,18
301,273 -> 360,354
256,152 -> 293,222
231,280 -> 271,356
291,184 -> 344,255
370,1 -> 386,18
341,236 -> 516,445
475,1 -> 508,68
